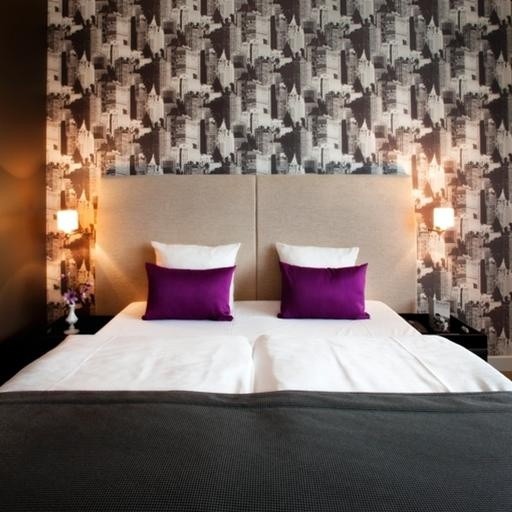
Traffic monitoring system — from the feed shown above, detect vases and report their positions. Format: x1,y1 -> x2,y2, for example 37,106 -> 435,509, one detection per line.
66,305 -> 80,328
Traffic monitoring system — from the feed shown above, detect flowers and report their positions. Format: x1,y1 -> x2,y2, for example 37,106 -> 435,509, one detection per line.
61,274 -> 91,305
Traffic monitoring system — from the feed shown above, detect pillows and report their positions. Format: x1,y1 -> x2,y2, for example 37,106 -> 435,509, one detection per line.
151,241 -> 242,314
143,263 -> 236,320
278,263 -> 370,319
276,243 -> 359,268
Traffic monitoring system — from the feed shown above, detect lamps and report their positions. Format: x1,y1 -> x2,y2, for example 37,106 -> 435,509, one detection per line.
428,208 -> 454,239
58,210 -> 79,235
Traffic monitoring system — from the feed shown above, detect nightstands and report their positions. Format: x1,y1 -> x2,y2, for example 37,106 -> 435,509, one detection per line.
43,315 -> 113,341
400,314 -> 487,360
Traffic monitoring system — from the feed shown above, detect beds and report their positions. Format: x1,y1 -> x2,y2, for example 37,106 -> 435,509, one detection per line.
0,175 -> 512,512
